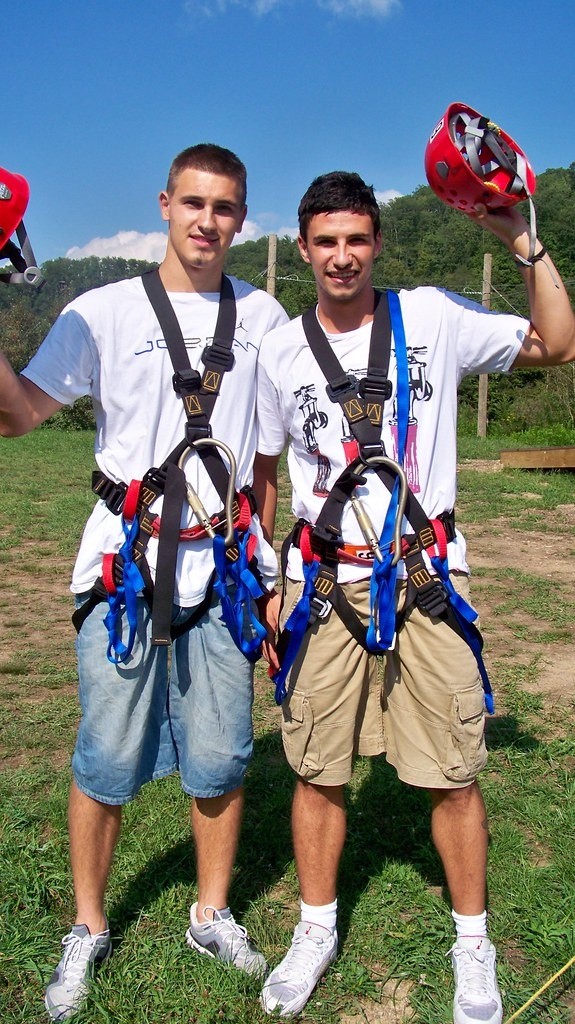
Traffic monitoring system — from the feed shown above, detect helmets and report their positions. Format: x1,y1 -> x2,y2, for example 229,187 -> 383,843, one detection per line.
0,167 -> 30,251
424,102 -> 537,214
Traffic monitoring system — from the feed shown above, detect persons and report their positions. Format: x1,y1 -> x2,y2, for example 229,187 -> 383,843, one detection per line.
253,171 -> 567,1024
0,140 -> 292,1024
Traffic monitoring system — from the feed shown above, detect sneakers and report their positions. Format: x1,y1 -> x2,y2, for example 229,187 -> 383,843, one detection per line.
445,935 -> 503,1024
185,901 -> 266,979
45,913 -> 112,1022
259,921 -> 338,1017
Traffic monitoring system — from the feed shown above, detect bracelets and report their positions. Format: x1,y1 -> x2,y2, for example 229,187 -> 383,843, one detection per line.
514,248 -> 549,267
